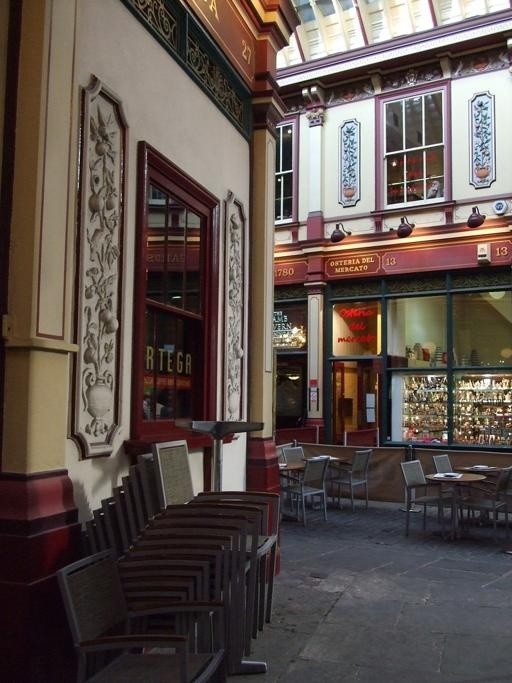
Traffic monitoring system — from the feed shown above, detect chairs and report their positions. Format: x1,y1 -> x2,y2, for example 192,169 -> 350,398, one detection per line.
57,440 -> 280,683
400,454 -> 512,546
278,447 -> 373,528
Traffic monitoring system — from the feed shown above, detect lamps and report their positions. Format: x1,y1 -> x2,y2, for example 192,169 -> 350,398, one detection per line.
397,216 -> 415,238
467,205 -> 486,228
331,223 -> 351,243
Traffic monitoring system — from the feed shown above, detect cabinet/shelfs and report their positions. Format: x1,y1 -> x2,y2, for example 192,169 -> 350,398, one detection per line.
402,374 -> 512,446
